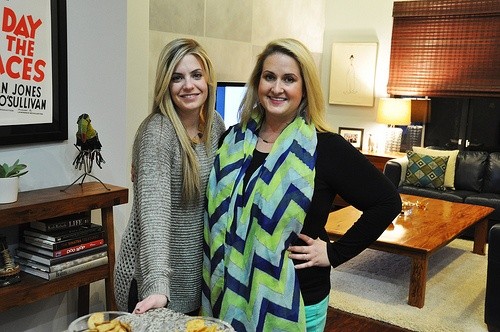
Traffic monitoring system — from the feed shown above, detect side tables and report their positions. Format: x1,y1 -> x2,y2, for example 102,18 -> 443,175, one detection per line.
332,151 -> 405,206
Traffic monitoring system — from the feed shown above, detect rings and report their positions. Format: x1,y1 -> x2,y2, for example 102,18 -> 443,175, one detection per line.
305,253 -> 308,260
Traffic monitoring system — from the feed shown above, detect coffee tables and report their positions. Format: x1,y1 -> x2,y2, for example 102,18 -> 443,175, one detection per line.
324,192 -> 495,309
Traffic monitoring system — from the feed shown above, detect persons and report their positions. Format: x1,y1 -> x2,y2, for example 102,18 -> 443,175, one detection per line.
113,38 -> 225,316
201,39 -> 401,332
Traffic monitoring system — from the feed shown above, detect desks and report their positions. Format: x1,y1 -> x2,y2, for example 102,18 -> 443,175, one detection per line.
0,180 -> 130,332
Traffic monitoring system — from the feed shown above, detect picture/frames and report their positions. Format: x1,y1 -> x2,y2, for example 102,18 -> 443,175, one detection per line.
338,126 -> 365,152
327,41 -> 379,108
0,0 -> 69,147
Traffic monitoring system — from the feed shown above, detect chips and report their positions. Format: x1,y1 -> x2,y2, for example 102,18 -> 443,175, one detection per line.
82,313 -> 133,332
184,319 -> 219,332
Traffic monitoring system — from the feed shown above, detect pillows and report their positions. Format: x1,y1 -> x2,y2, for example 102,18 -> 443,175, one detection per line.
402,151 -> 449,191
413,146 -> 460,190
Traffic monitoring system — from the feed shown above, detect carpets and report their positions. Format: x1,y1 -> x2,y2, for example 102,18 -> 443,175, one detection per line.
329,205 -> 500,332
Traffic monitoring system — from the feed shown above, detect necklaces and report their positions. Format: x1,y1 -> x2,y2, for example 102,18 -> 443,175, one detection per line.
196,132 -> 203,139
258,134 -> 274,143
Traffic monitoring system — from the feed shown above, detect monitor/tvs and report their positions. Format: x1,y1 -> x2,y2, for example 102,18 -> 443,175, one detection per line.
214,82 -> 248,132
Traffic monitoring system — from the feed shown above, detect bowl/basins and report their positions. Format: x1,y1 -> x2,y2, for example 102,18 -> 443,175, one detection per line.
67,311 -> 133,332
175,316 -> 235,332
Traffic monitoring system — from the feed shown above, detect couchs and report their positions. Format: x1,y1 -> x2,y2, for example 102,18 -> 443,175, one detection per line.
483,224 -> 500,332
384,146 -> 500,237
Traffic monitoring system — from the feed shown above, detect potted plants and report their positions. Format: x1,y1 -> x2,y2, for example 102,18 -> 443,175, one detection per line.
0,158 -> 28,203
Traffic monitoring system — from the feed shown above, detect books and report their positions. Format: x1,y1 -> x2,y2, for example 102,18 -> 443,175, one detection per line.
14,212 -> 108,280
